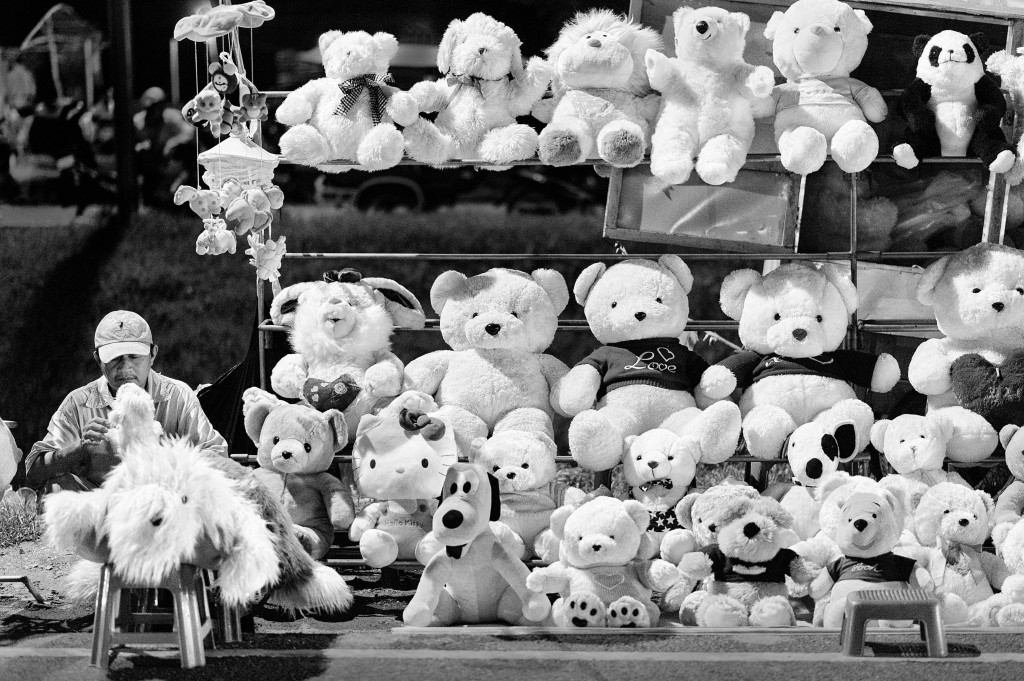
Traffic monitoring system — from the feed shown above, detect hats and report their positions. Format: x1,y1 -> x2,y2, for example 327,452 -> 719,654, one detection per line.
94,310 -> 152,364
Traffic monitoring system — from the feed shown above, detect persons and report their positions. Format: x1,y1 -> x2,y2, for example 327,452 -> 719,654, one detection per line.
26,308 -> 227,495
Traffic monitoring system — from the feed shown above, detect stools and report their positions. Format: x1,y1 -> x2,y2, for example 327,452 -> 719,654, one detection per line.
839,589 -> 947,658
90,563 -> 219,671
198,559 -> 253,643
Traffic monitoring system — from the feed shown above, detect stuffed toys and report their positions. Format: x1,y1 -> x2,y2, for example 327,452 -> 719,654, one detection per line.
280,27 -> 419,173
759,0 -> 890,178
170,50 -> 285,255
531,6 -> 664,172
409,10 -> 555,175
225,248 -> 1024,637
890,26 -> 1017,178
645,3 -> 775,189
58,388 -> 356,621
